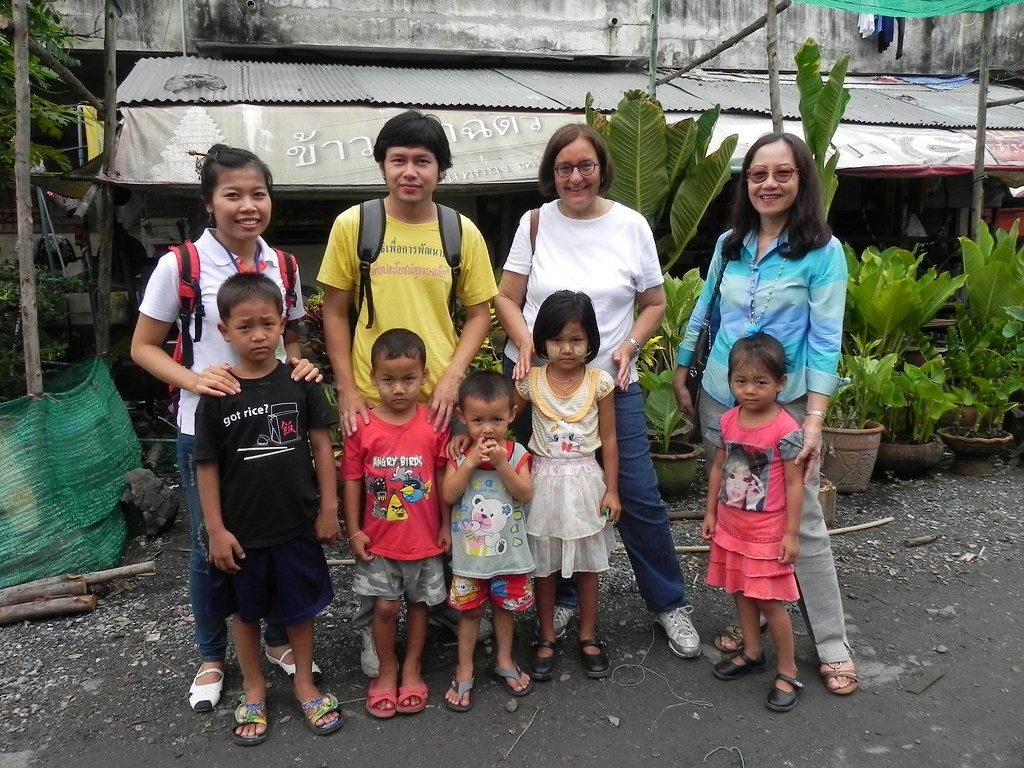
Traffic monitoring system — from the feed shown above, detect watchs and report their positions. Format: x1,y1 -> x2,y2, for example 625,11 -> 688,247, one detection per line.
624,337 -> 641,355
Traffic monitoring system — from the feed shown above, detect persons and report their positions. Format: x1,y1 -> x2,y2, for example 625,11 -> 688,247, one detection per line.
500,123 -> 701,660
678,134 -> 857,696
345,327 -> 452,719
128,144 -> 324,713
190,273 -> 344,744
700,333 -> 804,714
445,290 -> 620,678
445,369 -> 535,711
319,110 -> 500,676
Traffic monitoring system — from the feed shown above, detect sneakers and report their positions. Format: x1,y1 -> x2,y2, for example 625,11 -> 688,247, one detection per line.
429,604 -> 493,643
360,625 -> 399,677
534,606 -> 575,640
655,603 -> 702,658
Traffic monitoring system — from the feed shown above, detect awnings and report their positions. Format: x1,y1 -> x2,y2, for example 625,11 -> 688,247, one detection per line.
92,105 -> 1024,190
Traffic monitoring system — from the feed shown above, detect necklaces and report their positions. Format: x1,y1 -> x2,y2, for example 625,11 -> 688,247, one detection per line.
750,244 -> 791,322
549,365 -> 585,395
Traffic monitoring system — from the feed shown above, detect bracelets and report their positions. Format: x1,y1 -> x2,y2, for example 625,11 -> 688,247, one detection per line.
347,530 -> 362,542
804,410 -> 827,421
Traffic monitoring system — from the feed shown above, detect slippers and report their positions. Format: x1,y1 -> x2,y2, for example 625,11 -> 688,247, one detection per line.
366,678 -> 397,718
822,662 -> 859,695
396,685 -> 429,713
231,702 -> 267,746
486,661 -> 532,697
293,693 -> 344,736
714,618 -> 767,652
443,675 -> 473,712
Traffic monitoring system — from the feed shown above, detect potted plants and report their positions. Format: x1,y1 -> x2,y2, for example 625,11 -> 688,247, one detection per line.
633,267 -> 705,505
817,219 -> 1023,529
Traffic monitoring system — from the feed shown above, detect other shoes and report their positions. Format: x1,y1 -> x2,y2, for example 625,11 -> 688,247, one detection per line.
188,663 -> 224,712
265,648 -> 323,683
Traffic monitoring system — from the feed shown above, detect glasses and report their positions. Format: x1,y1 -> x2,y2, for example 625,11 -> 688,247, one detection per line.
553,160 -> 600,178
745,165 -> 799,183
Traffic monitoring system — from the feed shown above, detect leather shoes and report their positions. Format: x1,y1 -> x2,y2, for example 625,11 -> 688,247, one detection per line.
578,636 -> 611,678
765,670 -> 805,712
713,649 -> 766,680
528,640 -> 557,680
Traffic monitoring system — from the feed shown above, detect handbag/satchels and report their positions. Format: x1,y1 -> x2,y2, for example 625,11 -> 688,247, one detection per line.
685,304 -> 722,389
